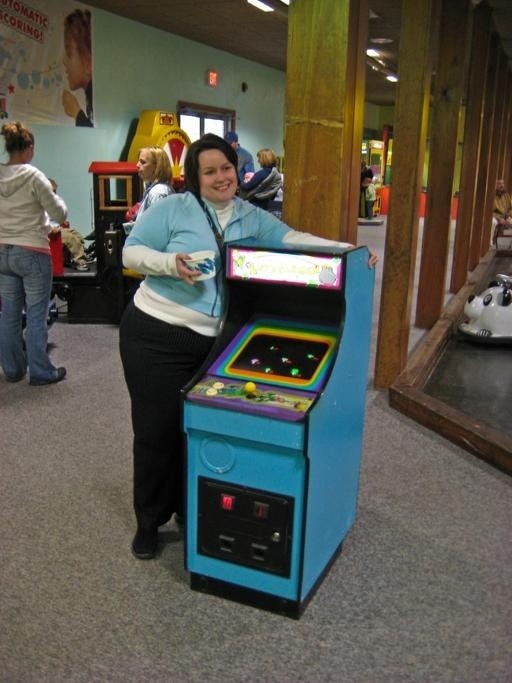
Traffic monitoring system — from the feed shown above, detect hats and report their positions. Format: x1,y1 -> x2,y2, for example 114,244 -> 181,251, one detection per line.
224,131 -> 238,144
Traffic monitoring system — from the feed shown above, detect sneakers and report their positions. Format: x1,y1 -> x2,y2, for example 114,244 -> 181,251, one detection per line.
48,366 -> 67,383
132,527 -> 158,560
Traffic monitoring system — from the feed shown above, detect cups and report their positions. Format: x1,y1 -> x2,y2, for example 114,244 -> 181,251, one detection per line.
123,222 -> 135,234
182,249 -> 217,281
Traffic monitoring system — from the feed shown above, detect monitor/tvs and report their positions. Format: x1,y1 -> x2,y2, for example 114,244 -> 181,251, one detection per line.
208,317 -> 338,392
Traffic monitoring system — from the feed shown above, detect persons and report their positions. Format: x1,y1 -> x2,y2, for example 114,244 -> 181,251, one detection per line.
128,147 -> 175,221
120,134 -> 378,560
0,124 -> 67,386
493,179 -> 512,241
364,178 -> 376,220
239,148 -> 281,210
46,177 -> 95,271
225,131 -> 255,181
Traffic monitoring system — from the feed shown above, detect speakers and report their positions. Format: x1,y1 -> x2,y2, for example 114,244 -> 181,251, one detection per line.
104,230 -> 122,267
